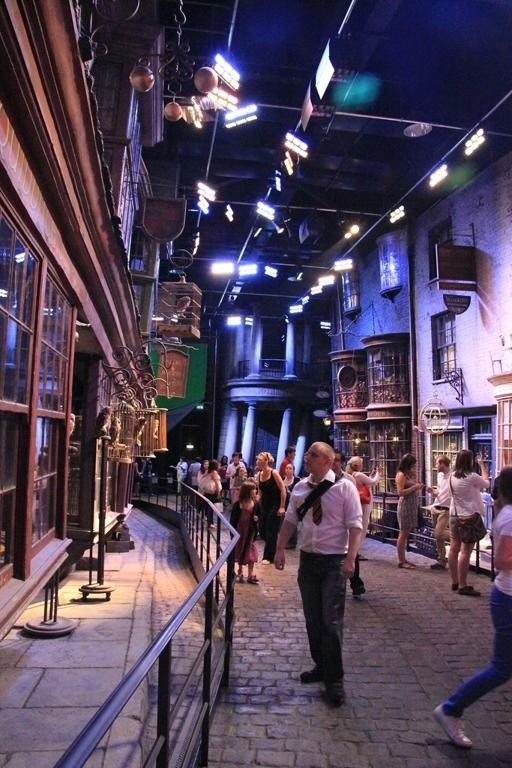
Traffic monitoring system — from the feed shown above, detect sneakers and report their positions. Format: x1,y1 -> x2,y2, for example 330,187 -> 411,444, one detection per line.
359,554 -> 368,561
352,592 -> 361,598
452,583 -> 480,596
237,573 -> 260,583
430,563 -> 442,569
433,703 -> 472,749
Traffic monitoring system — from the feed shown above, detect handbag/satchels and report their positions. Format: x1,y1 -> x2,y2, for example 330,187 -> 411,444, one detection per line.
356,483 -> 372,504
457,511 -> 487,543
199,479 -> 221,496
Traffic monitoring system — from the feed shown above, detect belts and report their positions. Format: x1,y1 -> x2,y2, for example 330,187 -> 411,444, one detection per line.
435,506 -> 449,510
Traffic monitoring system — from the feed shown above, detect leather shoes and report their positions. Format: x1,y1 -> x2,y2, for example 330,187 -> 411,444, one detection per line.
299,671 -> 324,682
327,681 -> 346,705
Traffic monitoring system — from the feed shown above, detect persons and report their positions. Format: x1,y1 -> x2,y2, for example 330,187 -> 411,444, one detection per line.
133,450 -> 188,496
275,441 -> 364,682
448,450 -> 490,596
333,451 -> 379,595
425,457 -> 451,568
189,447 -> 301,585
396,455 -> 422,567
433,467 -> 511,747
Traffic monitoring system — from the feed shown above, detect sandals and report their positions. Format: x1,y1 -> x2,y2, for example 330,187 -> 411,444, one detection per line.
399,561 -> 416,569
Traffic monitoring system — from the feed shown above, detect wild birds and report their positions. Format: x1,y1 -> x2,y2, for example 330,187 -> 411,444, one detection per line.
108,417 -> 122,444
176,296 -> 191,315
96,406 -> 111,430
134,416 -> 147,438
152,419 -> 160,437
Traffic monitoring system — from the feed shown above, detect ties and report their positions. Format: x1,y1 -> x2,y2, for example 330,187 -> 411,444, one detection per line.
312,485 -> 322,525
